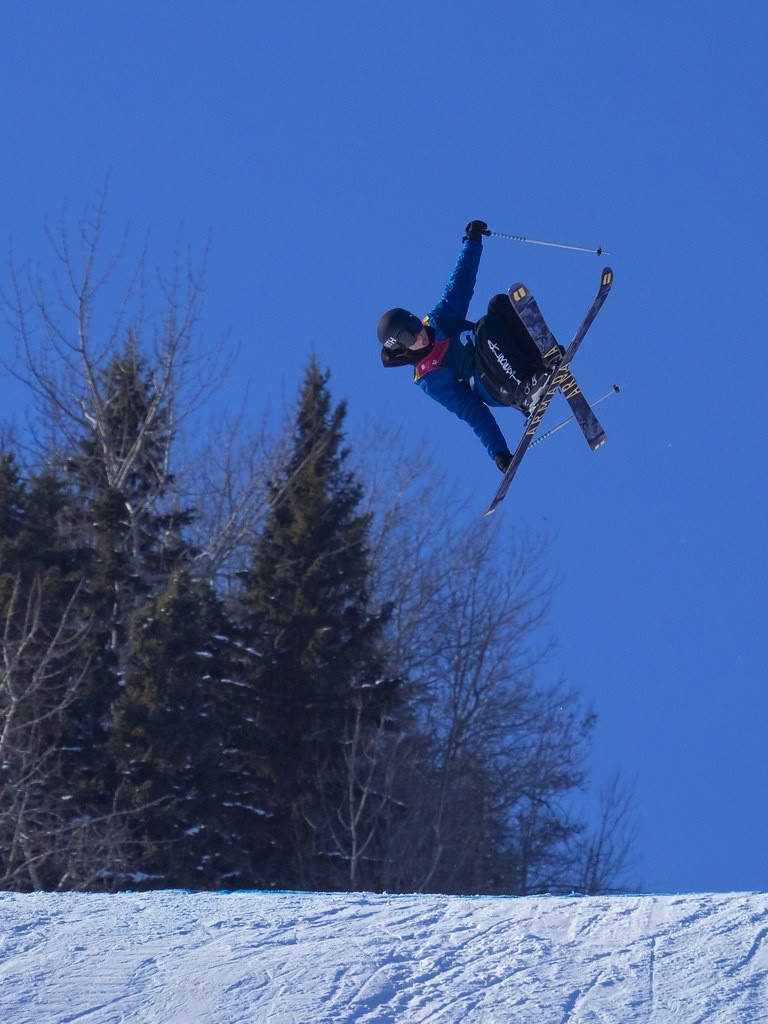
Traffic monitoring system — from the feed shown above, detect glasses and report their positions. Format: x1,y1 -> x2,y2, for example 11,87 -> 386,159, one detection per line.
398,313 -> 422,348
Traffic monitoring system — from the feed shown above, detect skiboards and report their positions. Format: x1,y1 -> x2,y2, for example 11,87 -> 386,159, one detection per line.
507,280 -> 610,453
478,265 -> 615,521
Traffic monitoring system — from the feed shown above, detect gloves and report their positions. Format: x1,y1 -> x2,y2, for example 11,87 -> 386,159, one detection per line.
465,219 -> 488,242
493,451 -> 514,474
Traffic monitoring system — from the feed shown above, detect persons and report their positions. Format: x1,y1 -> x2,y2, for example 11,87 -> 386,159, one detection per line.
377,220 -> 567,473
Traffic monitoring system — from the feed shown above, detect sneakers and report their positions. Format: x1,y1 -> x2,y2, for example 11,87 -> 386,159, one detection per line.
523,370 -> 553,414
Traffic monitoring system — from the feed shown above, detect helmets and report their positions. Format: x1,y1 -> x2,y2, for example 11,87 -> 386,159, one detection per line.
376,308 -> 412,351
